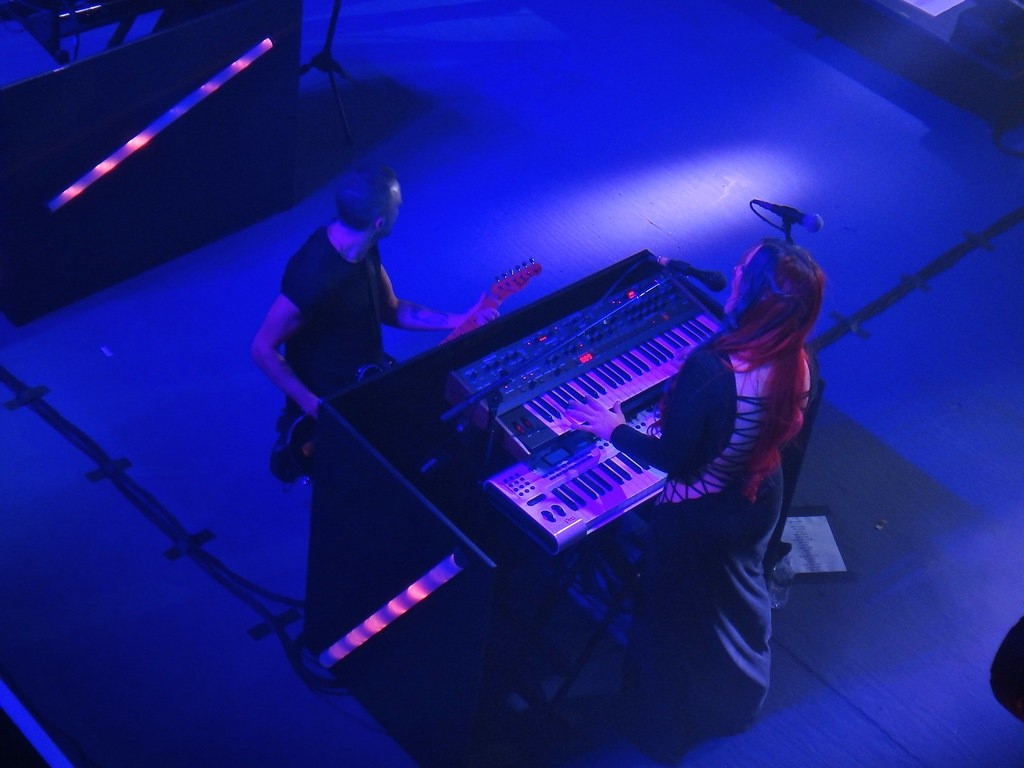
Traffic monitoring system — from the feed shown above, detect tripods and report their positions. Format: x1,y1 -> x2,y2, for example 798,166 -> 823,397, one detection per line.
297,1 -> 369,140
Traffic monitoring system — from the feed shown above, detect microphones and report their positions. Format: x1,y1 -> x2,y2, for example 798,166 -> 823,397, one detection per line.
751,195 -> 826,236
647,253 -> 728,293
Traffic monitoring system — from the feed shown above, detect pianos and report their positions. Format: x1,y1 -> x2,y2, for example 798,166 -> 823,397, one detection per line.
415,263 -> 739,740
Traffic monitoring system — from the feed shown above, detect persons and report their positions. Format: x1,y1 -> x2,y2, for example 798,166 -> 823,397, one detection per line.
566,239 -> 826,724
251,167 -> 500,481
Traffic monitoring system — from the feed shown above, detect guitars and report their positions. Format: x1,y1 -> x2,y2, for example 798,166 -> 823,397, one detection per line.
279,258 -> 544,486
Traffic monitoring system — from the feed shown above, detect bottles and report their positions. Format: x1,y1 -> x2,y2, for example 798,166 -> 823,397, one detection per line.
768,555 -> 795,609
